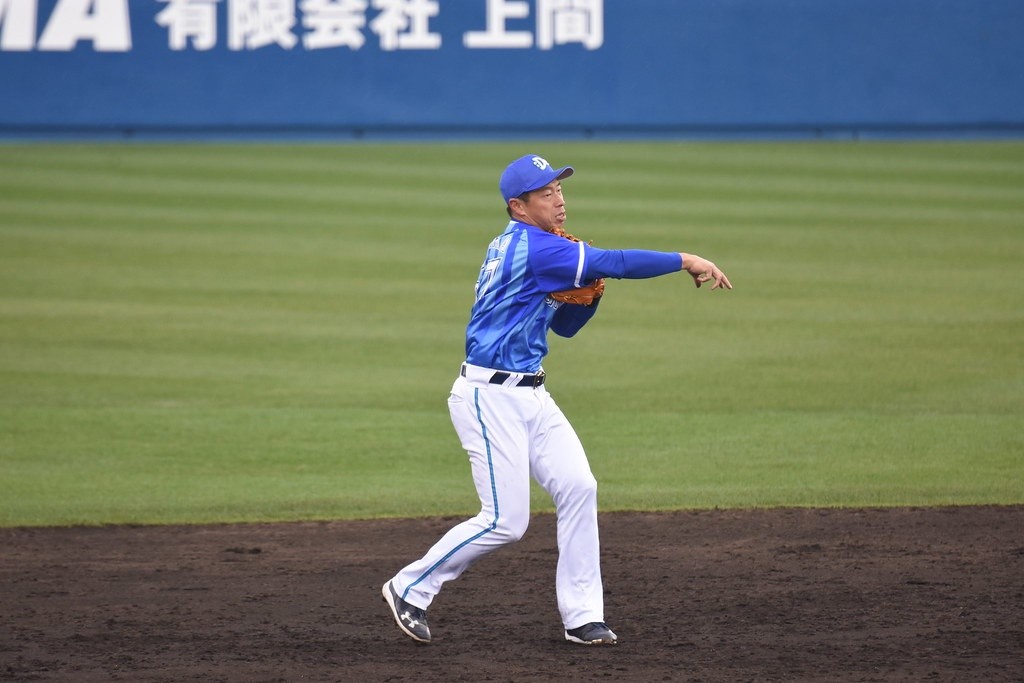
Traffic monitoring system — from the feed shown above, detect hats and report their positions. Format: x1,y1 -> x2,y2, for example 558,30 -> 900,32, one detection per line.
499,154 -> 574,206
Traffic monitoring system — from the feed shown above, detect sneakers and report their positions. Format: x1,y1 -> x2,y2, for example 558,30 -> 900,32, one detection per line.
564,623 -> 619,646
381,579 -> 431,643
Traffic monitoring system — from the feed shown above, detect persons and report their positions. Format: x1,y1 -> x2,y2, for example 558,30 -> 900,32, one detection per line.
380,154 -> 733,645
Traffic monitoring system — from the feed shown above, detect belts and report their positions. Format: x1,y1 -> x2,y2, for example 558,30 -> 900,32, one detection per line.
460,365 -> 547,389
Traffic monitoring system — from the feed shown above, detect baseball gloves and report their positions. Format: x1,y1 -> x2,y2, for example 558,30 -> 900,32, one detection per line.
546,226 -> 605,305
